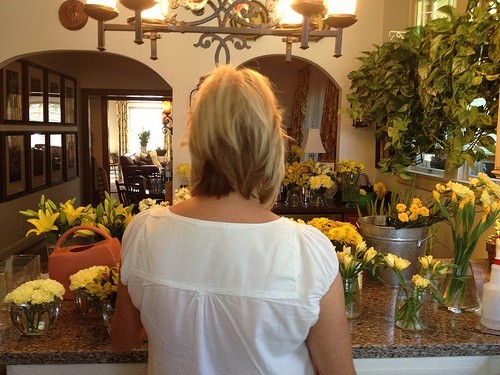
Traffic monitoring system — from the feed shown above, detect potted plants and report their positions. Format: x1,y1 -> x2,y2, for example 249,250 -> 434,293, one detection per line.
156,146 -> 167,156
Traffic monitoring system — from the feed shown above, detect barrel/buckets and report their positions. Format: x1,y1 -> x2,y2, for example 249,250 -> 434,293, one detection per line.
359,214 -> 432,285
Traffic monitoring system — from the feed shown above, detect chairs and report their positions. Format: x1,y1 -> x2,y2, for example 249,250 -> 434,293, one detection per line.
109,153 -> 120,176
110,180 -> 149,209
144,170 -> 167,204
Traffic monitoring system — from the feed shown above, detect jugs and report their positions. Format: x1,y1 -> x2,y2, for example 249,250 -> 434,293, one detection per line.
47,226 -> 122,302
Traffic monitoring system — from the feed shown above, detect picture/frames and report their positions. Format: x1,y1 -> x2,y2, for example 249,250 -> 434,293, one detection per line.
0,60 -> 80,202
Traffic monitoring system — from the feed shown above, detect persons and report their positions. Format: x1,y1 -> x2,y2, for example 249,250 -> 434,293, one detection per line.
111,64 -> 356,375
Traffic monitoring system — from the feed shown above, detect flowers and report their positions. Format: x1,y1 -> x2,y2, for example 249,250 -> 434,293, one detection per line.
275,144 -> 500,329
2,165 -> 193,333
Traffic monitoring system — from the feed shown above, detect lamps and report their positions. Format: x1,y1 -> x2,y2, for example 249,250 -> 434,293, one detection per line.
162,100 -> 173,135
301,126 -> 326,160
83,0 -> 358,67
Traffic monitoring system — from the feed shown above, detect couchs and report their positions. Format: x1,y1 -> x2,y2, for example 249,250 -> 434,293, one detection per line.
120,152 -> 169,188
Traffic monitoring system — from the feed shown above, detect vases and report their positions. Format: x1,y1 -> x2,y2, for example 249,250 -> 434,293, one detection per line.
393,285 -> 429,331
0,254 -> 41,311
308,188 -> 330,207
341,173 -> 360,207
75,289 -> 102,319
284,184 -> 304,206
10,303 -> 60,335
342,276 -> 361,319
46,234 -> 61,256
101,299 -> 115,335
439,260 -> 480,312
